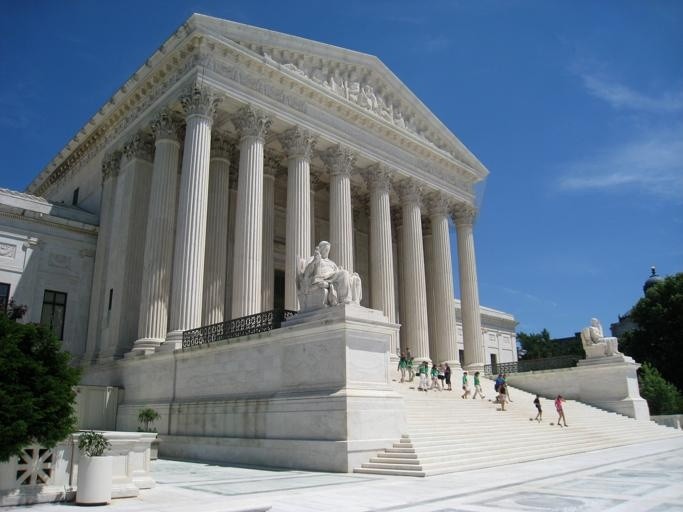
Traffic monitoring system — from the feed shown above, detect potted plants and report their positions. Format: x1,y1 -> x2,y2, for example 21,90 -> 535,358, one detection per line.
137,406 -> 161,460
76,431 -> 112,506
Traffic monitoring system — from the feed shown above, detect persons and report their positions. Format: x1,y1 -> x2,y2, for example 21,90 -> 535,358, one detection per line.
555,395 -> 567,426
533,394 -> 542,421
397,347 -> 512,412
304,241 -> 363,302
589,318 -> 620,355
